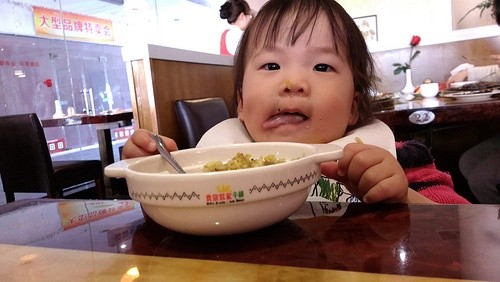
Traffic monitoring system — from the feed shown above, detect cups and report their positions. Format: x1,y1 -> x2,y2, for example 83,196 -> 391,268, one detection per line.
420,83 -> 439,98
67,107 -> 74,116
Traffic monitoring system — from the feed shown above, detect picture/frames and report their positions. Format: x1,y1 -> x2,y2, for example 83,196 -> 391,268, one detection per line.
352,15 -> 378,42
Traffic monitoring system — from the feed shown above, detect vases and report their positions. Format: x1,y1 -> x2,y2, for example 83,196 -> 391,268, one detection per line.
52,100 -> 65,119
400,69 -> 415,94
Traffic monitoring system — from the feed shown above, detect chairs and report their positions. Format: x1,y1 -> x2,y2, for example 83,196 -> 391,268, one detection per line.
0,113 -> 106,203
175,97 -> 230,149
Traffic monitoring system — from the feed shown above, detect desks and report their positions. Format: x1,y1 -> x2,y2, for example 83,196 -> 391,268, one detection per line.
41,111 -> 133,200
0,199 -> 500,282
372,96 -> 500,163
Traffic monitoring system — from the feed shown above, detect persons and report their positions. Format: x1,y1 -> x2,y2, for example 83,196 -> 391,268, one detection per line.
446,0 -> 500,205
121,0 -> 472,205
218,0 -> 251,56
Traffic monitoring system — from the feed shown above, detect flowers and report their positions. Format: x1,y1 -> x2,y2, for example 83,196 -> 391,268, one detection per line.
392,35 -> 421,75
44,79 -> 56,101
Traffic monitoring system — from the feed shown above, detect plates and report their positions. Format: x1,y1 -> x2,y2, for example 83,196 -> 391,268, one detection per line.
444,89 -> 500,101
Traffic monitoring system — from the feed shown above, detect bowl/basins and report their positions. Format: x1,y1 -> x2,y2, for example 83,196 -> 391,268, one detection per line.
103,141 -> 346,236
449,80 -> 480,88
